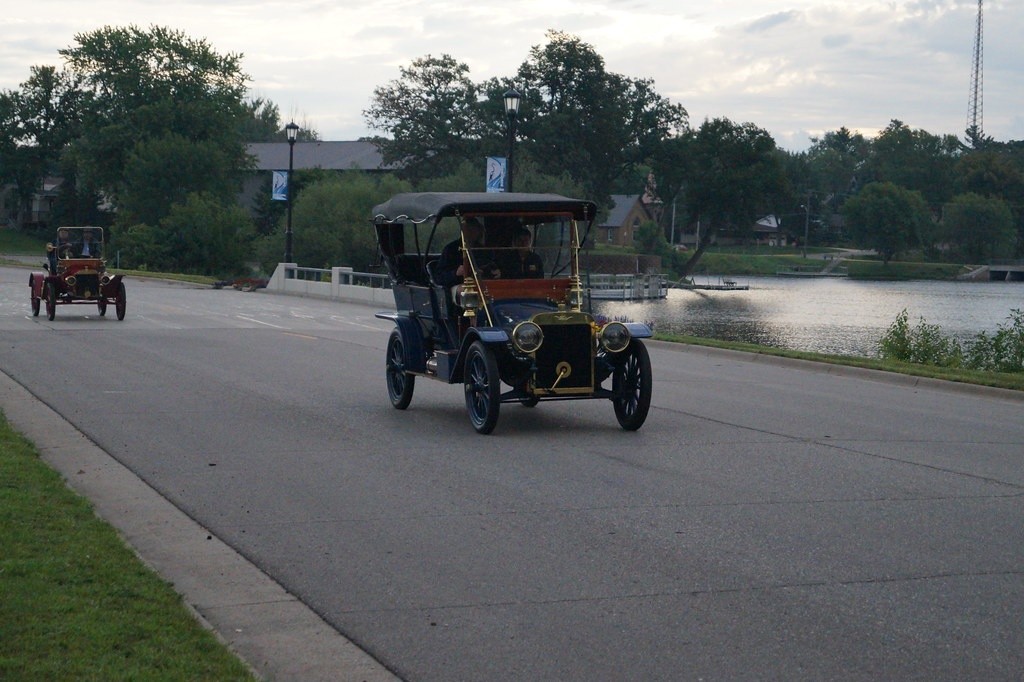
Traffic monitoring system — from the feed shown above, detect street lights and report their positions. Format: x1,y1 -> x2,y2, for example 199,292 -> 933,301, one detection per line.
800,204 -> 809,258
284,118 -> 300,263
501,82 -> 523,192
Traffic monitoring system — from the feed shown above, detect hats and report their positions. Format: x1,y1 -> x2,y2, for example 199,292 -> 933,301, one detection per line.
82,225 -> 96,234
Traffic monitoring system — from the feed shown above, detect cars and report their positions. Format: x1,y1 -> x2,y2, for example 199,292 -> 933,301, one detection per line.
366,191 -> 654,437
28,225 -> 127,321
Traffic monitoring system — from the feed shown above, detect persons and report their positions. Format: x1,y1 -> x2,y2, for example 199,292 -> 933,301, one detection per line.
73,225 -> 101,259
498,228 -> 544,280
435,216 -> 502,327
46,229 -> 79,271
